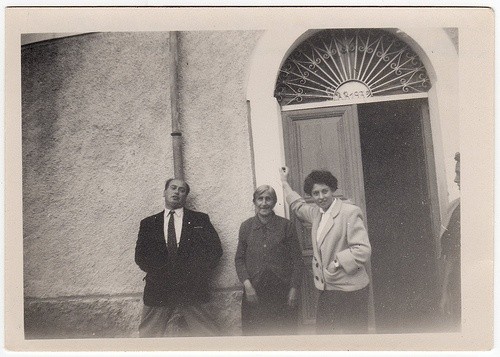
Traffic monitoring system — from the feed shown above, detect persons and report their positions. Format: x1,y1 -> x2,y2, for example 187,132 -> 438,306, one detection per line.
280,166 -> 372,335
134,179 -> 223,337
437,151 -> 459,314
233,185 -> 303,336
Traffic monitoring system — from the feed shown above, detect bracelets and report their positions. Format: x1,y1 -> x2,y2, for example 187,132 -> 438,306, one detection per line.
333,260 -> 340,269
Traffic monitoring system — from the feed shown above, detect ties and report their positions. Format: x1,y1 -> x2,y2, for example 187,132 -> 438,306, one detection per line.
167,212 -> 177,260
